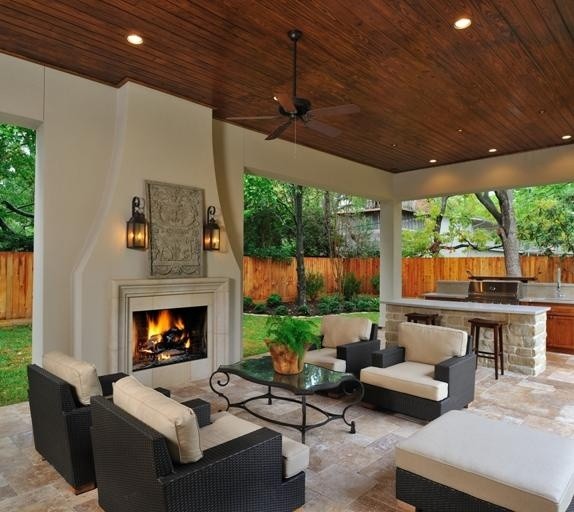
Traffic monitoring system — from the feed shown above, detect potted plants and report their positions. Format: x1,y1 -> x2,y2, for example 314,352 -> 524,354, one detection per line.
263,310 -> 322,375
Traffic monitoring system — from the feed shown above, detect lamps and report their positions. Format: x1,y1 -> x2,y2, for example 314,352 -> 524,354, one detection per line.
127,197 -> 149,249
203,206 -> 220,251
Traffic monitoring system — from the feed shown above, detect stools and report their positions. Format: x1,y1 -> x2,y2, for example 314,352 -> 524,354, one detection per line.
405,313 -> 438,325
468,318 -> 508,380
394,409 -> 574,512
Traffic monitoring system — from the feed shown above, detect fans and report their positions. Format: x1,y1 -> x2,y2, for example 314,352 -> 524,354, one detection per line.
226,29 -> 360,141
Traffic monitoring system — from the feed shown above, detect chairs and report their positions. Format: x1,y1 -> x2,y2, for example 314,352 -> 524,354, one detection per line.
27,364 -> 170,495
360,336 -> 476,421
309,324 -> 381,393
89,395 -> 310,512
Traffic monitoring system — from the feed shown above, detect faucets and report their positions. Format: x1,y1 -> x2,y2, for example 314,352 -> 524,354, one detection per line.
556,267 -> 563,297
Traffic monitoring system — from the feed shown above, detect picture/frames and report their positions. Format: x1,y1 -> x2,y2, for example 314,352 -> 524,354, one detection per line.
143,179 -> 208,279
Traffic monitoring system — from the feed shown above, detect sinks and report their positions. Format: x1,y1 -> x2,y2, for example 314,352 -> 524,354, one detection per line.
544,297 -> 574,301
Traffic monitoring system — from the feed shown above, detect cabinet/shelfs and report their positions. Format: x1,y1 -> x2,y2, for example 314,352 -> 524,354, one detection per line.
520,302 -> 574,350
